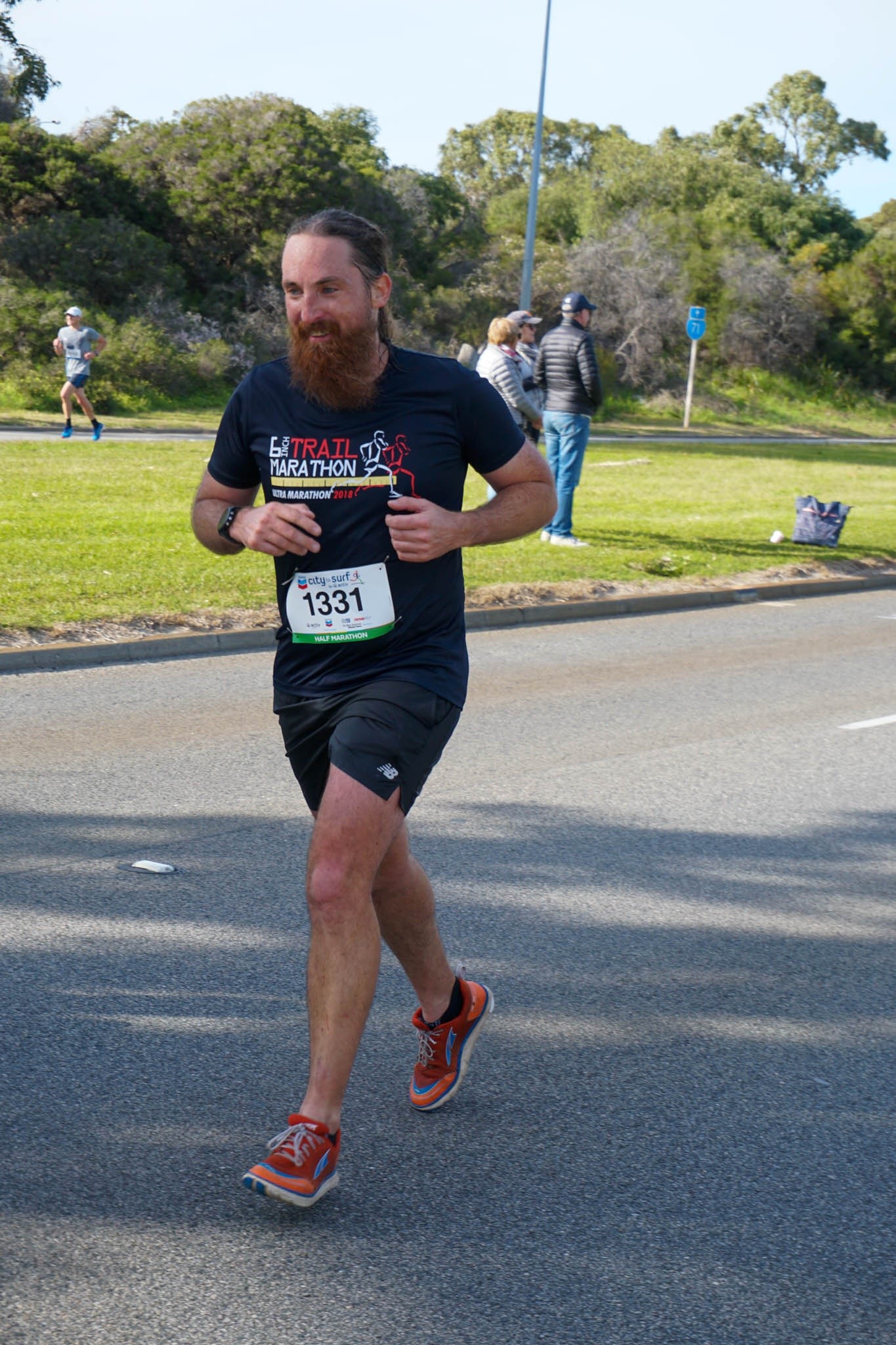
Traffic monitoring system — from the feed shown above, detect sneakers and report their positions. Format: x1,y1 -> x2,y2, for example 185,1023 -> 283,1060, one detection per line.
62,425 -> 73,437
92,423 -> 105,441
409,964 -> 494,1111
241,1114 -> 341,1208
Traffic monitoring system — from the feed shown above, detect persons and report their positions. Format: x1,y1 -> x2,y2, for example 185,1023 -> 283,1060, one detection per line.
506,309 -> 545,448
533,292 -> 600,546
189,209 -> 559,1209
52,306 -> 107,442
474,318 -> 543,504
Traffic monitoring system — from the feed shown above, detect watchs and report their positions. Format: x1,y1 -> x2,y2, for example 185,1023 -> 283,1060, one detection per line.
217,504 -> 246,544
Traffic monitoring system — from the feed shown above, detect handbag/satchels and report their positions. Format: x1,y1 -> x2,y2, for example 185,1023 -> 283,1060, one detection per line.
791,495 -> 856,548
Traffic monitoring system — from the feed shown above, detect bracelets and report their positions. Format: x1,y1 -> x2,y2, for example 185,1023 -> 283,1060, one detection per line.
93,349 -> 99,357
53,345 -> 58,348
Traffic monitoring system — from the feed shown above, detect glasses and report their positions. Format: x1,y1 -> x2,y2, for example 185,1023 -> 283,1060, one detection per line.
527,324 -> 537,330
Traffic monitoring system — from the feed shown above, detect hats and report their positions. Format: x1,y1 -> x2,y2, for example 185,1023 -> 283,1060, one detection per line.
507,310 -> 543,326
64,306 -> 82,318
561,293 -> 597,313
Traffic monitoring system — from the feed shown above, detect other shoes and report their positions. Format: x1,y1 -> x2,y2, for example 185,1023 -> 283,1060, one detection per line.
541,530 -> 590,547
